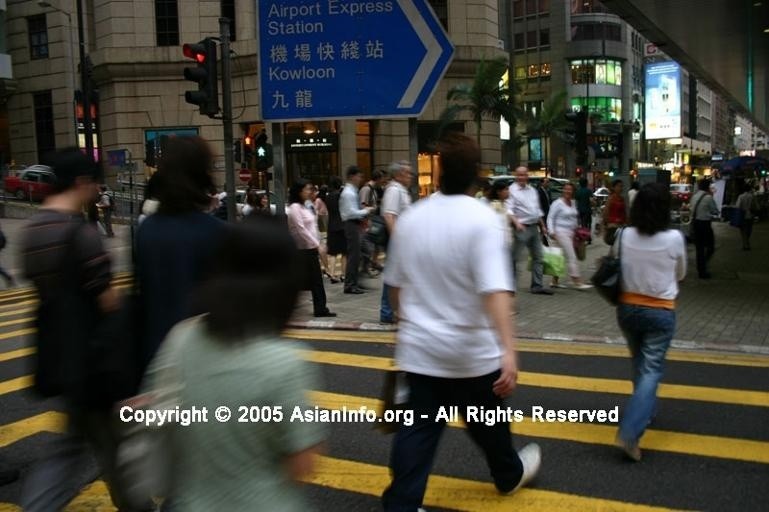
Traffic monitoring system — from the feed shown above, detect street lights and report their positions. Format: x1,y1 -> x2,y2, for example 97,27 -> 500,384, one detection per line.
35,0 -> 77,96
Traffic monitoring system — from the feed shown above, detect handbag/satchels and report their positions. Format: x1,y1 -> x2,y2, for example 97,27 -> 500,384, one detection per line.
526,236 -> 566,278
573,238 -> 586,261
589,224 -> 626,307
685,220 -> 699,245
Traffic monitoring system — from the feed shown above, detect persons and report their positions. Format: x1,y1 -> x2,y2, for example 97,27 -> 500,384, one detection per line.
605,180 -> 688,461
93,158 -> 768,326
136,208 -> 334,512
373,133 -> 544,511
17,145 -> 156,511
134,130 -> 241,366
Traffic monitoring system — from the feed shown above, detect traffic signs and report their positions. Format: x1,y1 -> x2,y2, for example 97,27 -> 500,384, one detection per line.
255,0 -> 457,124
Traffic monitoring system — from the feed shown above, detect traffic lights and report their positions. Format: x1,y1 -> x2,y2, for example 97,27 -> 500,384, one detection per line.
575,167 -> 583,177
243,136 -> 253,163
180,39 -> 220,118
255,133 -> 268,172
563,110 -> 587,156
234,140 -> 242,163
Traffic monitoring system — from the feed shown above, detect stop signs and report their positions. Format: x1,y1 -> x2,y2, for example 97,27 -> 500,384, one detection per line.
238,168 -> 250,181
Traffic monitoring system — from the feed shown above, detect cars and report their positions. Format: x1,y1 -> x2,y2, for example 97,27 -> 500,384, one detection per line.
487,172 -> 695,211
208,184 -> 290,222
0,160 -> 59,205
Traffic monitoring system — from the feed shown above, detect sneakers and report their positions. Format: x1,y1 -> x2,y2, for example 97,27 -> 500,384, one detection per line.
314,310 -> 337,318
497,441 -> 542,497
615,432 -> 643,463
530,287 -> 555,295
550,281 -> 569,289
573,282 -> 593,290
380,315 -> 398,326
342,282 -> 368,295
